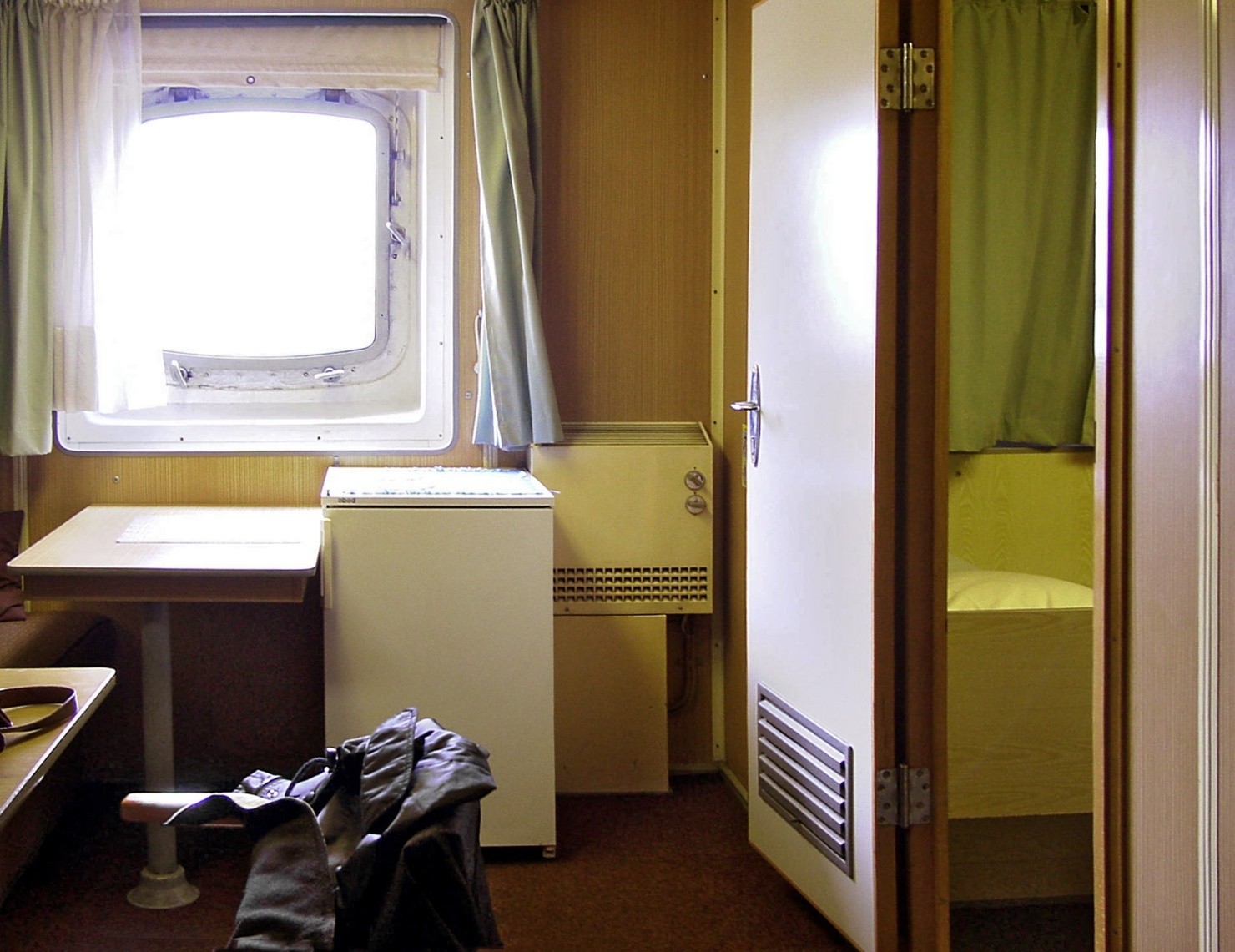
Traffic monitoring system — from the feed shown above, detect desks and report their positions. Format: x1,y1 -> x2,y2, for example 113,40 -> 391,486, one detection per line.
0,667 -> 116,836
6,505 -> 323,911
